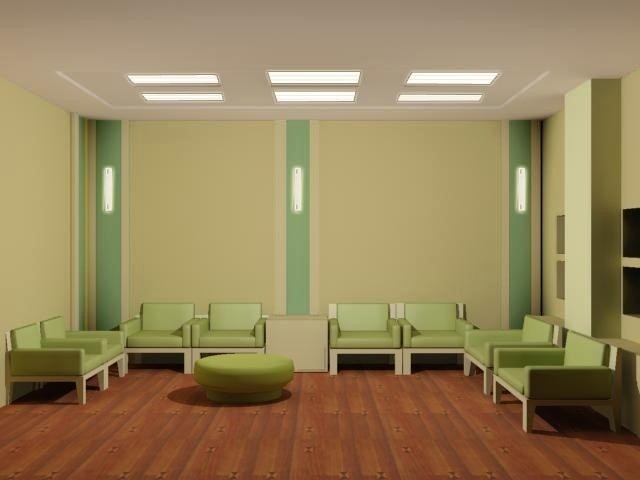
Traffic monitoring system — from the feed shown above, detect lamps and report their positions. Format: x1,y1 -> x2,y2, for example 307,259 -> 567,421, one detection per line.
516,167 -> 527,213
104,166 -> 114,212
293,166 -> 303,213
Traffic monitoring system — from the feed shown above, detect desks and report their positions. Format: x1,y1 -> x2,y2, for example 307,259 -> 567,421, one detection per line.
265,314 -> 328,373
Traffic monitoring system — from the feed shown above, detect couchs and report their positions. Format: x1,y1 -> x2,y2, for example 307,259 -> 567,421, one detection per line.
192,302 -> 269,375
327,302 -> 403,376
464,312 -> 563,396
396,301 -> 478,377
40,317 -> 126,393
492,329 -> 623,434
5,322 -> 106,406
117,302 -> 201,375
192,353 -> 295,404
